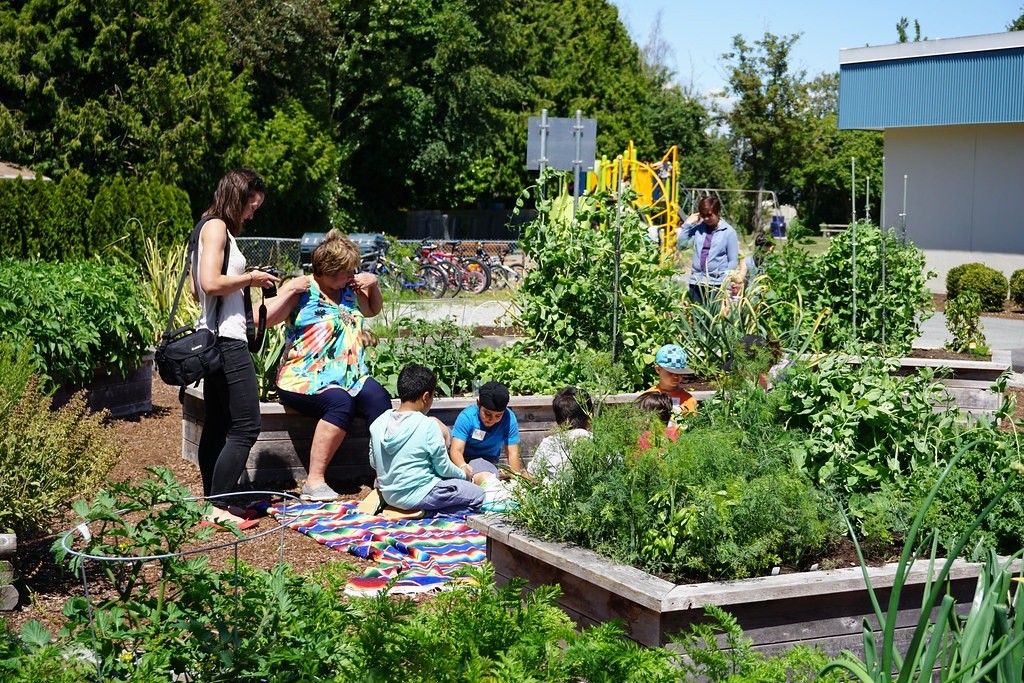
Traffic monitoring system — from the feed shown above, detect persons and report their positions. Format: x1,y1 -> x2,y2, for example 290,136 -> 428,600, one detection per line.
253,229 -> 393,501
730,232 -> 776,301
190,169 -> 280,530
646,344 -> 699,429
625,156 -> 673,242
523,386 -> 623,488
626,391 -> 680,461
678,196 -> 738,311
369,363 -> 486,520
450,381 -> 520,485
721,334 -> 796,394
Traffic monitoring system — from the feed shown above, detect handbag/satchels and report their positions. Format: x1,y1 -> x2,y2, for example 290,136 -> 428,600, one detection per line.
154,327 -> 225,386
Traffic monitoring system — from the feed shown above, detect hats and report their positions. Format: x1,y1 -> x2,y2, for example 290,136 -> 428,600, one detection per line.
655,344 -> 695,374
479,382 -> 510,412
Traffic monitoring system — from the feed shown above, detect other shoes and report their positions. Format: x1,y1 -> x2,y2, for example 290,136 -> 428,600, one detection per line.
216,505 -> 258,519
382,505 -> 425,519
201,518 -> 260,532
299,483 -> 345,502
356,488 -> 386,515
374,479 -> 380,488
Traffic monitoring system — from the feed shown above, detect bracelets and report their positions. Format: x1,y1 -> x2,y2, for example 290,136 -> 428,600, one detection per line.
459,464 -> 467,469
246,273 -> 253,287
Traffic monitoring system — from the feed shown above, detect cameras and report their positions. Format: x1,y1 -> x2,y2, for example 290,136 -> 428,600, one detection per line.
260,266 -> 287,299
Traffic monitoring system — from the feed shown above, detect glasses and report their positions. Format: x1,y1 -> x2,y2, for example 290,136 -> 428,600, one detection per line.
700,212 -> 714,218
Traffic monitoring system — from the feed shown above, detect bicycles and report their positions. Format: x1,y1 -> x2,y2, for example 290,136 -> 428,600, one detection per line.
245,263 -> 300,289
355,236 -> 528,298
